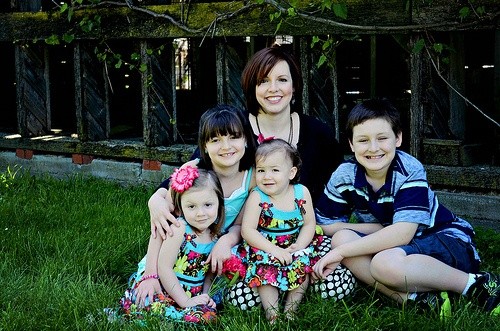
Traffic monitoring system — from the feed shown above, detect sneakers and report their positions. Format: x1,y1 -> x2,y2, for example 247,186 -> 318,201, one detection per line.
467,270 -> 500,316
415,291 -> 451,322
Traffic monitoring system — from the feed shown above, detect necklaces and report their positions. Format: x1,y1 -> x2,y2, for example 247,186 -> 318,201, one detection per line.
255,116 -> 295,145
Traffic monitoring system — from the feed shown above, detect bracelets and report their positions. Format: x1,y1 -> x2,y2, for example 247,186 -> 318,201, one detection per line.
141,274 -> 158,279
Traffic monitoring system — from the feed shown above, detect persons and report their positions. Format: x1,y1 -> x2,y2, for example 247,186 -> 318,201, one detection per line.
118,47 -> 498,322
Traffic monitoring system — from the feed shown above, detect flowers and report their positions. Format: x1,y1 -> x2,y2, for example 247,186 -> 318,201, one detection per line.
169,164 -> 199,192
257,133 -> 275,145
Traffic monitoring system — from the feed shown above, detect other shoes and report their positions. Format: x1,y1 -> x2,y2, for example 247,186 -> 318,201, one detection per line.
266,316 -> 283,326
285,313 -> 298,322
